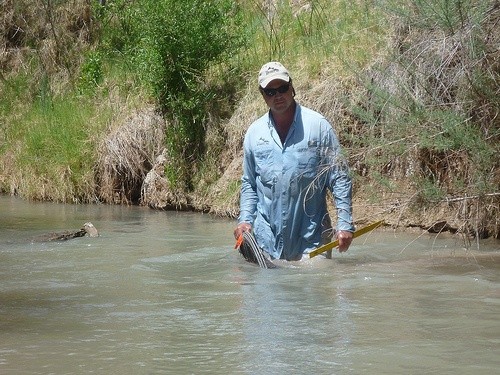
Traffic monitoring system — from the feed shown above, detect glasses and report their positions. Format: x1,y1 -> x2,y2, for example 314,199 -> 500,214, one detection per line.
261,84 -> 290,96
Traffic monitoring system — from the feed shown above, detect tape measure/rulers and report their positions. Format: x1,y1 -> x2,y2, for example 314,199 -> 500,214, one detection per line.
309,219 -> 385,258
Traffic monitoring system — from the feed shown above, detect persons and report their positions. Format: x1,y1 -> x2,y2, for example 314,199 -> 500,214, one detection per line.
233,61 -> 357,261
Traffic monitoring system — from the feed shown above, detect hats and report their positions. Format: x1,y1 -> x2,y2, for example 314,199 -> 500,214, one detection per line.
259,61 -> 290,88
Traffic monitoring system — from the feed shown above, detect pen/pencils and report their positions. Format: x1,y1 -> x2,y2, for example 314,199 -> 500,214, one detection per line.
312,140 -> 314,144
